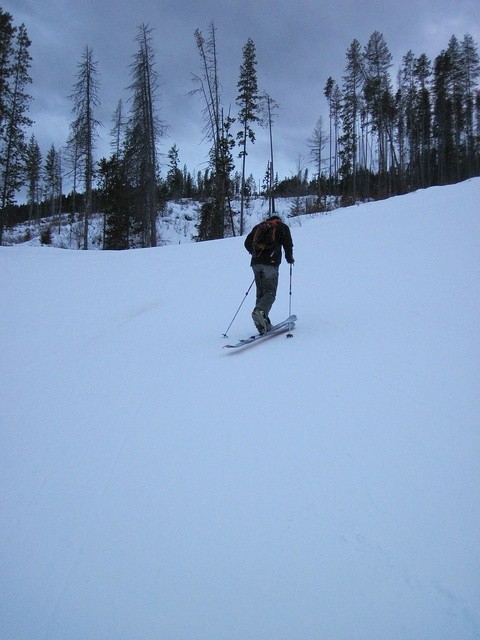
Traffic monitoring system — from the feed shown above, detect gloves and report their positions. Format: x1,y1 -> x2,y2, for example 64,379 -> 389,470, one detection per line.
287,259 -> 294,263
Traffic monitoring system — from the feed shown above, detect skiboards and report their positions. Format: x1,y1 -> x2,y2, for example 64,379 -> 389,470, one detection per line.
224,314 -> 297,348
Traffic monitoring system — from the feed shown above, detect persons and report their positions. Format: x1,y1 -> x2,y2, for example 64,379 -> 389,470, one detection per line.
244,212 -> 295,335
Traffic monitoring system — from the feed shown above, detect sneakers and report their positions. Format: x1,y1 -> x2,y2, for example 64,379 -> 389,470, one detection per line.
251,307 -> 273,334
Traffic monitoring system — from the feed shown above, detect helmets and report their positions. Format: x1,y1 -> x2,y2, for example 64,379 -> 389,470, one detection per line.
269,212 -> 285,221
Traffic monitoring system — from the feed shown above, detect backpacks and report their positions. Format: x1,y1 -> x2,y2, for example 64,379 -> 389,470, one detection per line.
252,219 -> 280,257
